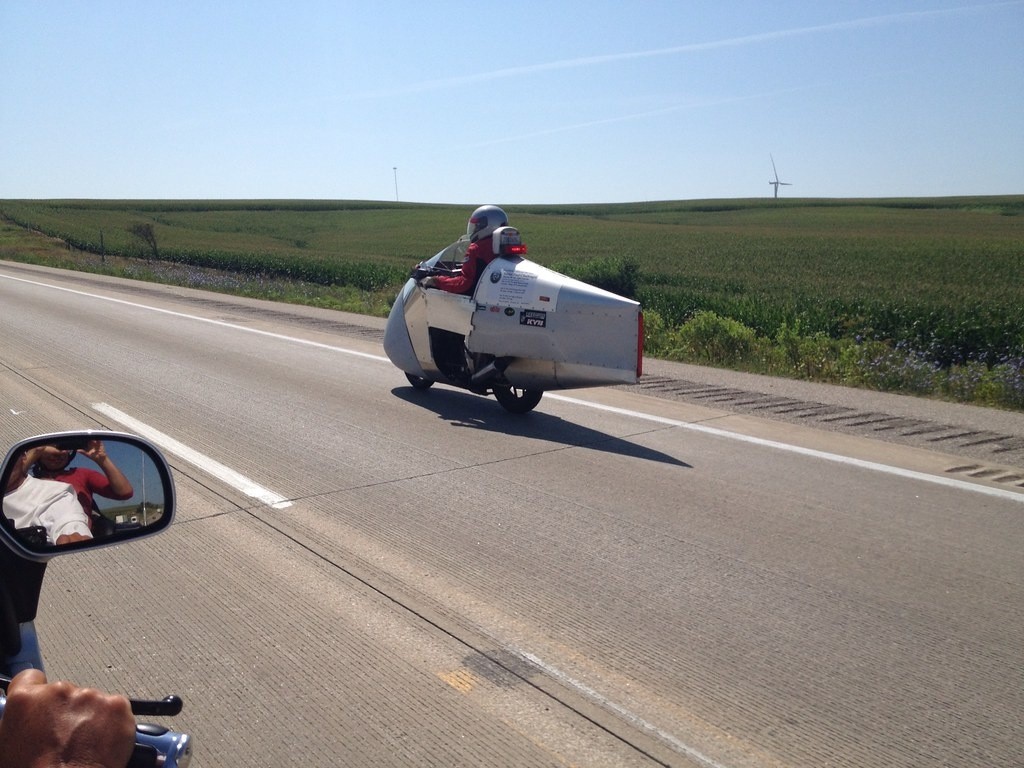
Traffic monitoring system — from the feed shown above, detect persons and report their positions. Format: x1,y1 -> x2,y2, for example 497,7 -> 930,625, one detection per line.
431,205 -> 509,387
0,668 -> 137,768
2,451 -> 93,546
21,439 -> 133,534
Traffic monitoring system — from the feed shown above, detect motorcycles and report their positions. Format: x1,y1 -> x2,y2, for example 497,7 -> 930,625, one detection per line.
382,225 -> 643,413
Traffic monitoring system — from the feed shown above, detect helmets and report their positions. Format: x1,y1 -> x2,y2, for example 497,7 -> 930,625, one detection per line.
467,204 -> 509,243
